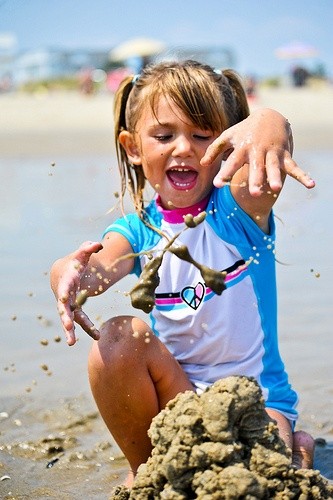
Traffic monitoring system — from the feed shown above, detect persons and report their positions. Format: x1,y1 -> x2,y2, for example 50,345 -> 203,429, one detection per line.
0,60 -> 312,104
49,61 -> 315,489
